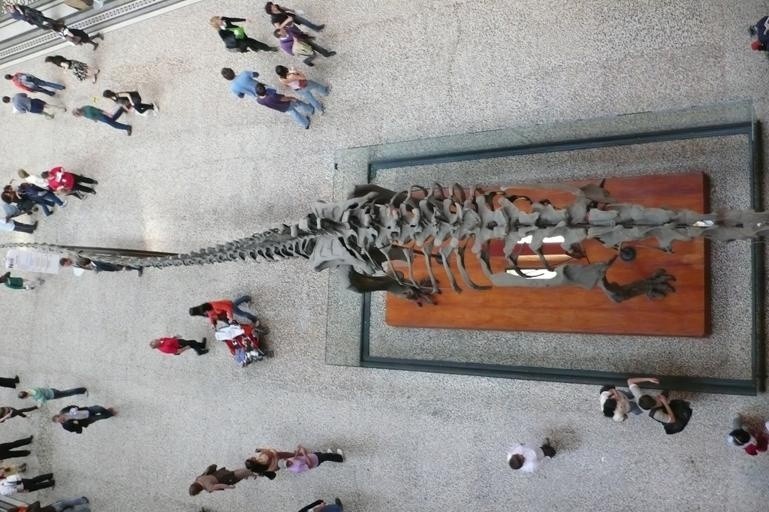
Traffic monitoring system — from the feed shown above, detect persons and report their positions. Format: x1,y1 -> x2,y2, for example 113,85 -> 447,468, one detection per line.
627,375 -> 678,426
748,12 -> 769,51
727,411 -> 768,457
597,385 -> 642,423
508,436 -> 557,474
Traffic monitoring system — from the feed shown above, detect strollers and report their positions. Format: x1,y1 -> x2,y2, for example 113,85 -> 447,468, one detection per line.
212,320 -> 274,368
